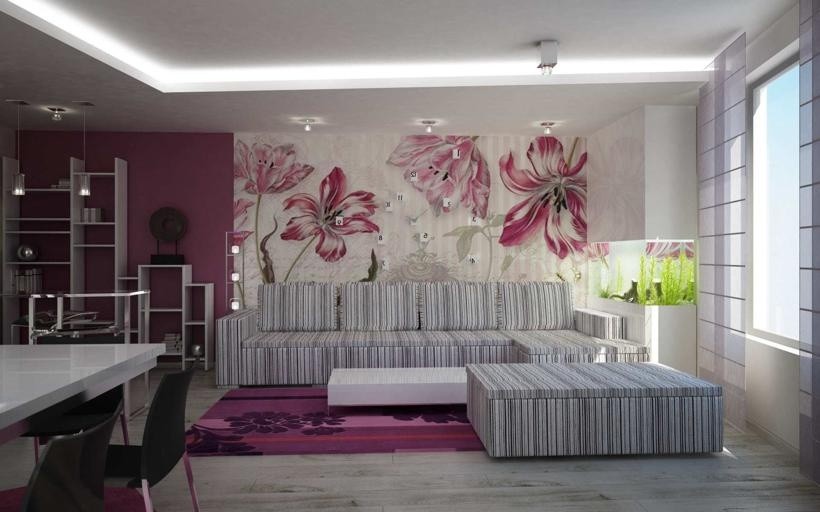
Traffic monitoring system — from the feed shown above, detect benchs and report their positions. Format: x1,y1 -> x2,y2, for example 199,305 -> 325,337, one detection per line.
464,363 -> 724,458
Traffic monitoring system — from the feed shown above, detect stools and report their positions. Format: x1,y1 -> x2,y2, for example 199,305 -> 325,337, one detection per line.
10,319 -> 28,344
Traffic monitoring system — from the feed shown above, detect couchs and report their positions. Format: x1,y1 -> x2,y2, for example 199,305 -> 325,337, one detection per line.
213,282 -> 648,387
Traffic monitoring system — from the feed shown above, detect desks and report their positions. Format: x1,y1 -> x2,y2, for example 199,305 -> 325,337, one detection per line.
0,341 -> 167,444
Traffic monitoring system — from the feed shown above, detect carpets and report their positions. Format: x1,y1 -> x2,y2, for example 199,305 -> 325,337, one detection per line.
173,386 -> 483,457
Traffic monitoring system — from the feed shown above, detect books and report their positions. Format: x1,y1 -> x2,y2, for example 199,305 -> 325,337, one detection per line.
79,207 -> 102,224
161,332 -> 182,355
9,268 -> 44,295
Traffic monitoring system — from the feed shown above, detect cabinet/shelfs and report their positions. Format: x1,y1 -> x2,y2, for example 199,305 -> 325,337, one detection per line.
119,264 -> 213,371
28,290 -> 151,423
0,156 -> 128,344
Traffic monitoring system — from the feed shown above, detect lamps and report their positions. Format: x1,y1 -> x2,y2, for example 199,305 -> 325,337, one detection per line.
52,108 -> 62,121
304,119 -> 312,131
77,104 -> 91,196
544,122 -> 552,135
537,40 -> 558,76
11,103 -> 25,196
225,232 -> 244,315
425,122 -> 433,133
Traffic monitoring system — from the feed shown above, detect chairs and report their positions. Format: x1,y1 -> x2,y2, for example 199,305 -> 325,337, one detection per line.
21,404 -> 124,512
106,370 -> 202,512
20,336 -> 130,463
